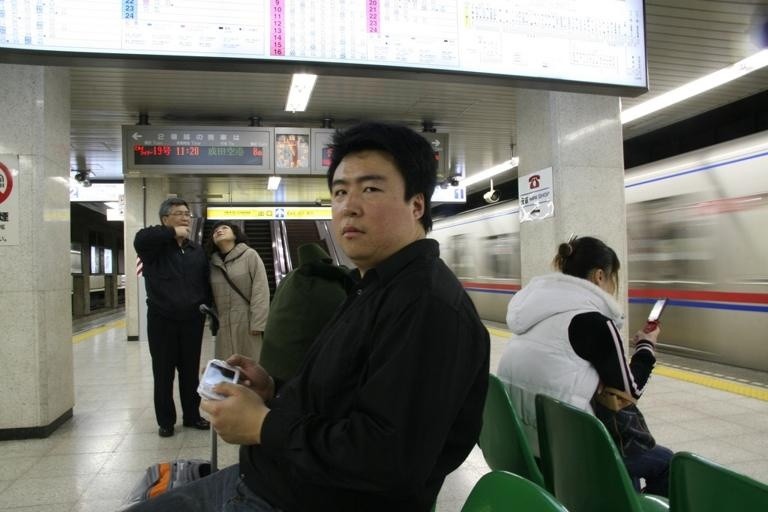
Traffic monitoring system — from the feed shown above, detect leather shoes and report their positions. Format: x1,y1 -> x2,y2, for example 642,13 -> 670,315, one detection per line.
157,423 -> 174,437
182,417 -> 210,431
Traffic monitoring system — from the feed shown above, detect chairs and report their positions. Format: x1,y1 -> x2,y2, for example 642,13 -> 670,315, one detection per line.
472,375 -> 546,487
664,448 -> 765,510
530,391 -> 667,510
461,467 -> 568,512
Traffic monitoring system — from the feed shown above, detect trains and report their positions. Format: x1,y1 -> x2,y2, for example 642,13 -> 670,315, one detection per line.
427,129 -> 768,374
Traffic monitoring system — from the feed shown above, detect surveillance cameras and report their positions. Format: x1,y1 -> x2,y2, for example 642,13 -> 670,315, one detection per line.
483,190 -> 500,203
76,175 -> 91,187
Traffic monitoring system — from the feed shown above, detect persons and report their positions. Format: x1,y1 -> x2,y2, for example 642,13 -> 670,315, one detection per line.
120,119 -> 491,512
209,221 -> 270,364
495,234 -> 674,501
133,198 -> 215,437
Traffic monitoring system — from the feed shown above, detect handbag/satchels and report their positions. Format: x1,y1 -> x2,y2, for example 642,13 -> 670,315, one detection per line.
259,242 -> 359,385
591,387 -> 656,458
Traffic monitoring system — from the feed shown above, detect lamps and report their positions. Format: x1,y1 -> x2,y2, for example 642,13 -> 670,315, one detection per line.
453,143 -> 518,191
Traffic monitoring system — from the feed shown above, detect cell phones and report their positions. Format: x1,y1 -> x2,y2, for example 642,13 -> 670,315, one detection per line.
644,297 -> 668,333
198,359 -> 241,400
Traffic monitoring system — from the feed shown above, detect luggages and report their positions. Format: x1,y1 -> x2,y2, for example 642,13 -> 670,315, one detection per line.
116,426 -> 218,511
